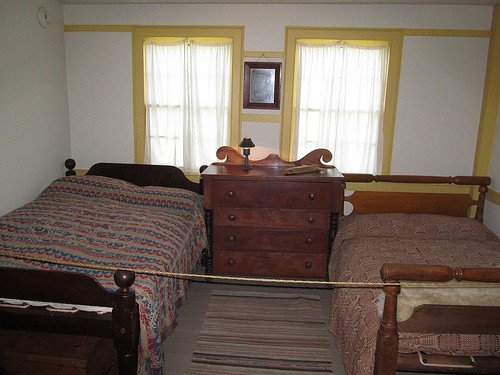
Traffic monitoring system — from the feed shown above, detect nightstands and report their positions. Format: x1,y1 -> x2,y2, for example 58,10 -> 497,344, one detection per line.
201,148 -> 346,286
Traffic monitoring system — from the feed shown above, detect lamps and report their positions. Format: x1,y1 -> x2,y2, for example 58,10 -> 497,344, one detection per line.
239,137 -> 255,170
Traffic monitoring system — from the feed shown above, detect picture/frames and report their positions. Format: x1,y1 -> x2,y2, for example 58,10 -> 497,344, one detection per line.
243,62 -> 282,110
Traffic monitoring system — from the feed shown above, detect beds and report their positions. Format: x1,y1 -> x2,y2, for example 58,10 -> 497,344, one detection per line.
0,159 -> 208,375
328,173 -> 500,375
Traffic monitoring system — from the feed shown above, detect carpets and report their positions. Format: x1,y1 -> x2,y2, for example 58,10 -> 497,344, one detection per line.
190,290 -> 334,375
